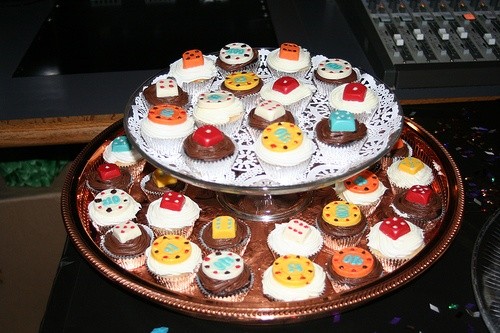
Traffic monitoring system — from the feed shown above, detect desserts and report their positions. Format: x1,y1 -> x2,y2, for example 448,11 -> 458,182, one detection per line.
133,42 -> 380,184
86,135 -> 445,301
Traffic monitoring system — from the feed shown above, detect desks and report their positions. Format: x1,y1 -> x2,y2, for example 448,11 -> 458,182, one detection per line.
36,98 -> 500,333
